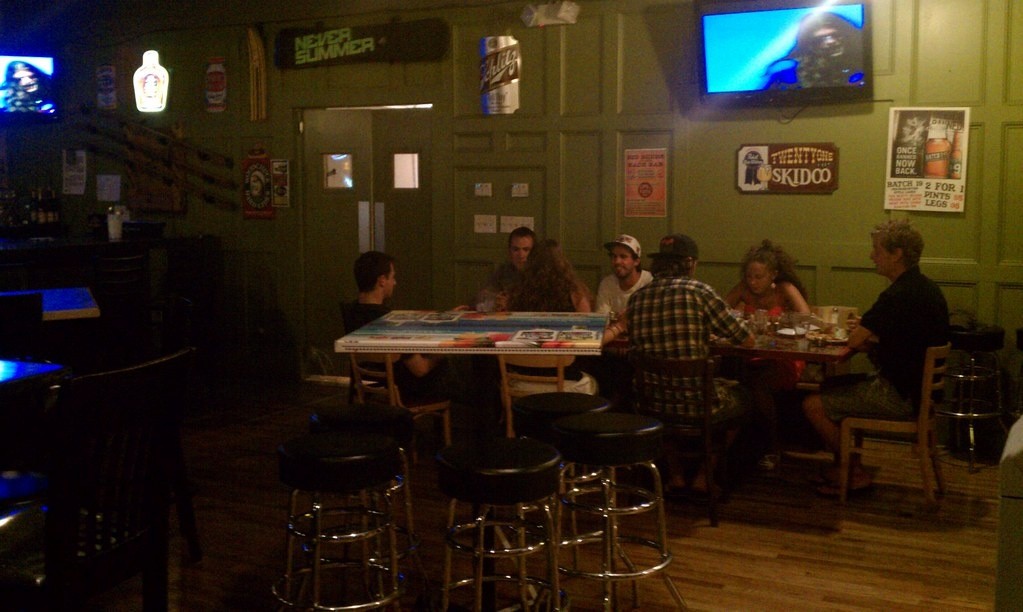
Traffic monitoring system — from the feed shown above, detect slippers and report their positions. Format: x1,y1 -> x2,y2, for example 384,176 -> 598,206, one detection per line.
809,475 -> 878,500
662,480 -> 727,506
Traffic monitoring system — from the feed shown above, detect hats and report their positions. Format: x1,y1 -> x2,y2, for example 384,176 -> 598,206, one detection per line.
647,233 -> 700,260
604,234 -> 642,259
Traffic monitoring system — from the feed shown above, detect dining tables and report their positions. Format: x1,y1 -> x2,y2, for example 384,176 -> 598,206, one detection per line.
603,313 -> 852,362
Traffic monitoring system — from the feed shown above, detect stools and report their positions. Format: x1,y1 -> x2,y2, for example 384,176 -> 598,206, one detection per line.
946,324 -> 1013,472
268,390 -> 691,611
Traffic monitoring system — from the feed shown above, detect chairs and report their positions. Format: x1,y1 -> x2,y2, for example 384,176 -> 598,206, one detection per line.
0,347 -> 190,612
497,348 -> 597,435
837,343 -> 954,509
90,253 -> 151,351
626,349 -> 748,525
352,351 -> 451,462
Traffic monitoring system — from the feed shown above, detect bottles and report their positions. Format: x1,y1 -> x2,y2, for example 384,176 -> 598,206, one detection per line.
27,190 -> 60,224
830,308 -> 841,324
95,54 -> 117,111
205,57 -> 228,113
924,124 -> 952,177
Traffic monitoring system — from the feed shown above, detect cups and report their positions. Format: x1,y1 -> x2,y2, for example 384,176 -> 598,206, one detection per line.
107,213 -> 122,243
751,310 -> 767,342
792,312 -> 812,336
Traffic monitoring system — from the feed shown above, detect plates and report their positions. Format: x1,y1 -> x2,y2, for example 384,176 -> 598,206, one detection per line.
805,331 -> 848,344
776,325 -> 821,335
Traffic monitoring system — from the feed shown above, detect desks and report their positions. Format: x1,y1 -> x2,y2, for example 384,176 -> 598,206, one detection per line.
0,352 -> 66,390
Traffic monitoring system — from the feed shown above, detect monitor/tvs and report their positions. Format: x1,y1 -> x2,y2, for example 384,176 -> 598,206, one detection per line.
698,0 -> 874,107
0,49 -> 66,125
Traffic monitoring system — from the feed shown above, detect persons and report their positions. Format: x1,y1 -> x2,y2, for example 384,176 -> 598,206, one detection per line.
762,11 -> 866,89
458,227 -> 655,399
625,232 -> 757,501
342,249 -> 491,469
801,219 -> 949,490
720,238 -> 812,472
